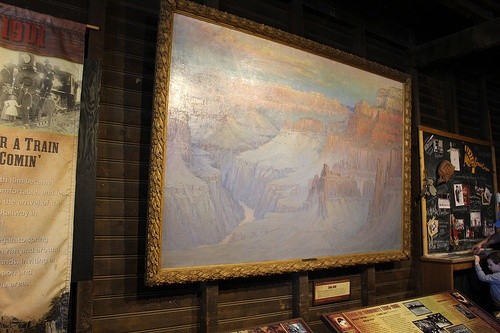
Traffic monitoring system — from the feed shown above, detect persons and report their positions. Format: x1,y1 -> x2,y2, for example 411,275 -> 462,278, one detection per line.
473,252 -> 499,319
471,218 -> 500,254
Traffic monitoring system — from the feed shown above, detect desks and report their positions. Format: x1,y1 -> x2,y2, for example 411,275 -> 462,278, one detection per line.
419,247 -> 495,297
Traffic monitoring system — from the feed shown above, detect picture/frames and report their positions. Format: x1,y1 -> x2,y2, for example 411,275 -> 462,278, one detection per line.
141,0 -> 412,286
417,124 -> 500,258
